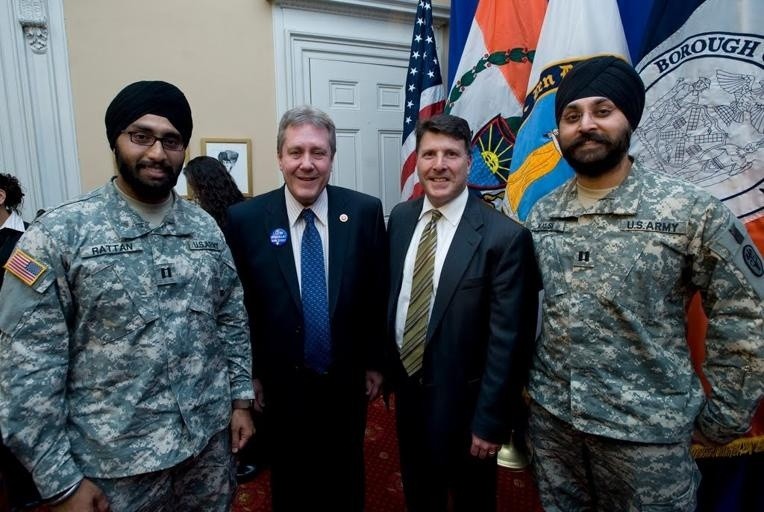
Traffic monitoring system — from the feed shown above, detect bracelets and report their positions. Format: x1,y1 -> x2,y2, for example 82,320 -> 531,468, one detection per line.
46,483 -> 80,506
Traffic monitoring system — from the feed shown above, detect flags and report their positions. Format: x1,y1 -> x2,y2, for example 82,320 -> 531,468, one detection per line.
500,0 -> 654,225
444,1 -> 549,211
400,1 -> 445,202
633,1 -> 764,457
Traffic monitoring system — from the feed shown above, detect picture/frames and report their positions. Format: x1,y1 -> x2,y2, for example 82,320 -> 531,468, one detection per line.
201,138 -> 253,198
173,146 -> 194,201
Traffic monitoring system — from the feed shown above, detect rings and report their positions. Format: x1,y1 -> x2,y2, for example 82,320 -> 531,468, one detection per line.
488,450 -> 498,455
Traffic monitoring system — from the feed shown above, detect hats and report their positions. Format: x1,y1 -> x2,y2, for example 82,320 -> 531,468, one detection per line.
555,56 -> 646,132
105,81 -> 193,150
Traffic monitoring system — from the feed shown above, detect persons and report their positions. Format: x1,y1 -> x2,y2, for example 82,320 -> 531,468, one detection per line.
227,105 -> 390,512
182,156 -> 245,229
0,82 -> 255,512
378,116 -> 538,512
525,56 -> 764,512
1,172 -> 31,284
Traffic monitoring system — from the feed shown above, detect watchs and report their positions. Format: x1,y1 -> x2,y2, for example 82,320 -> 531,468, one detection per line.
233,399 -> 253,409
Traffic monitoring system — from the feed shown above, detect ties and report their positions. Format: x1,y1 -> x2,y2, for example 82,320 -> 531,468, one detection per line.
299,209 -> 331,372
399,211 -> 443,376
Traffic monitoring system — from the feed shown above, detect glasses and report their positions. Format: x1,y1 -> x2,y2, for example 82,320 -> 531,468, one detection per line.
121,131 -> 185,152
